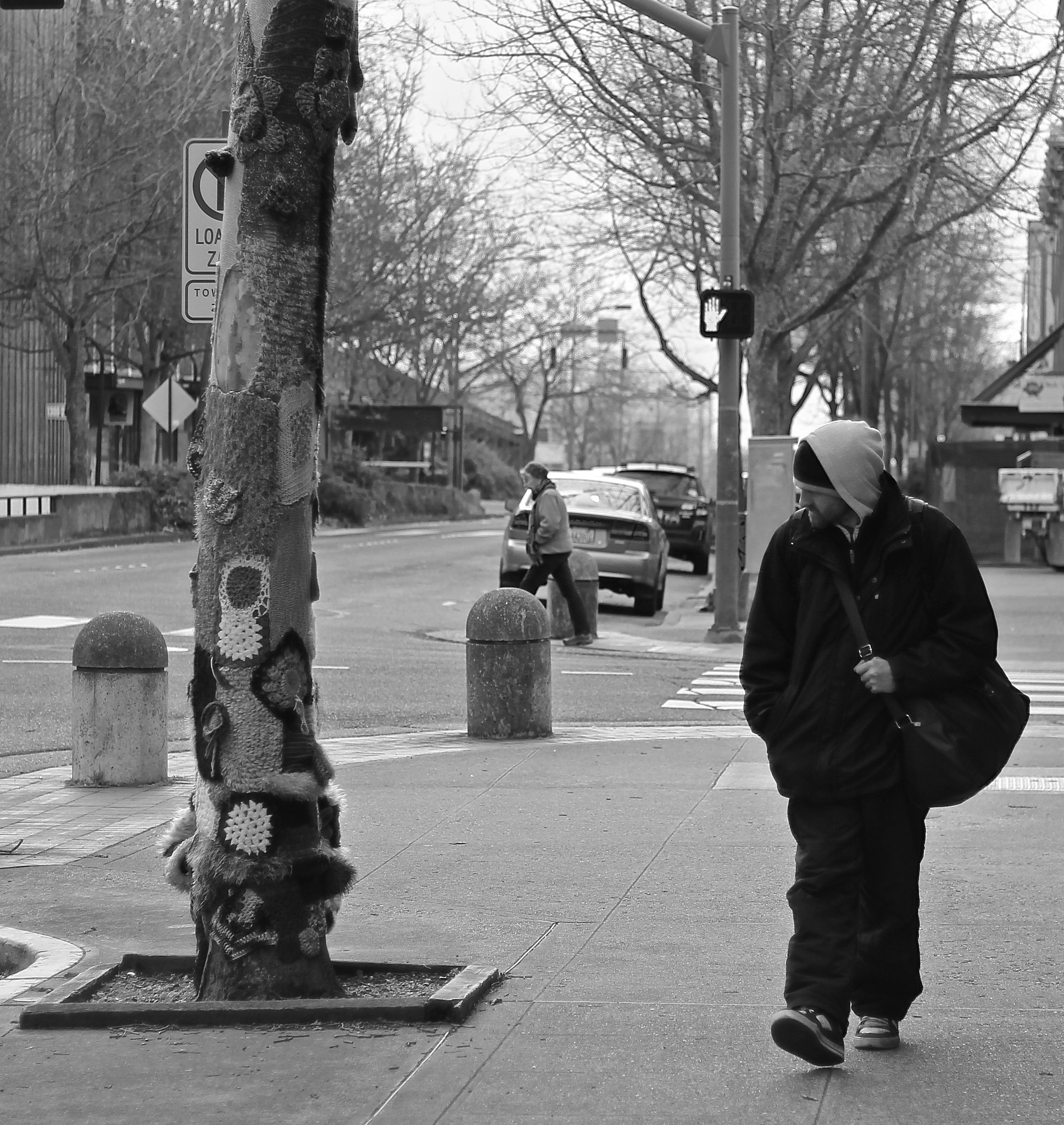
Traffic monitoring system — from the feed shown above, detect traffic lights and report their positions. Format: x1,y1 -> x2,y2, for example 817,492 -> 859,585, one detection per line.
700,289 -> 755,340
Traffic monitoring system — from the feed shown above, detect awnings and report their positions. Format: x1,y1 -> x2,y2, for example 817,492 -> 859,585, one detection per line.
958,401 -> 1064,430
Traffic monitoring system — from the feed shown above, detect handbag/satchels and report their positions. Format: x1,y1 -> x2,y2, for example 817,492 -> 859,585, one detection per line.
886,656 -> 1028,807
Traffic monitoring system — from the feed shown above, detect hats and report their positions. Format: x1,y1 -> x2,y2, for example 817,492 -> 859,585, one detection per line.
793,441 -> 840,497
525,462 -> 549,479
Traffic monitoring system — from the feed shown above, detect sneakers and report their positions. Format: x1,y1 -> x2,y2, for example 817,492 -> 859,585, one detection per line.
854,1013 -> 901,1049
562,633 -> 593,646
770,1008 -> 844,1066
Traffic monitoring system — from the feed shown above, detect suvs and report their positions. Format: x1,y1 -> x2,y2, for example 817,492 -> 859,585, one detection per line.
611,460 -> 715,577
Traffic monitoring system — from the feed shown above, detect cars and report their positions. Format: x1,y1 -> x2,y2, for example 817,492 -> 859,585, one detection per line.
498,471 -> 670,618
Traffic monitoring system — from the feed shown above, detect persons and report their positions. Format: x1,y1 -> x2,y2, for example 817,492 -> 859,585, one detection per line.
518,462 -> 595,647
739,410 -> 999,1069
